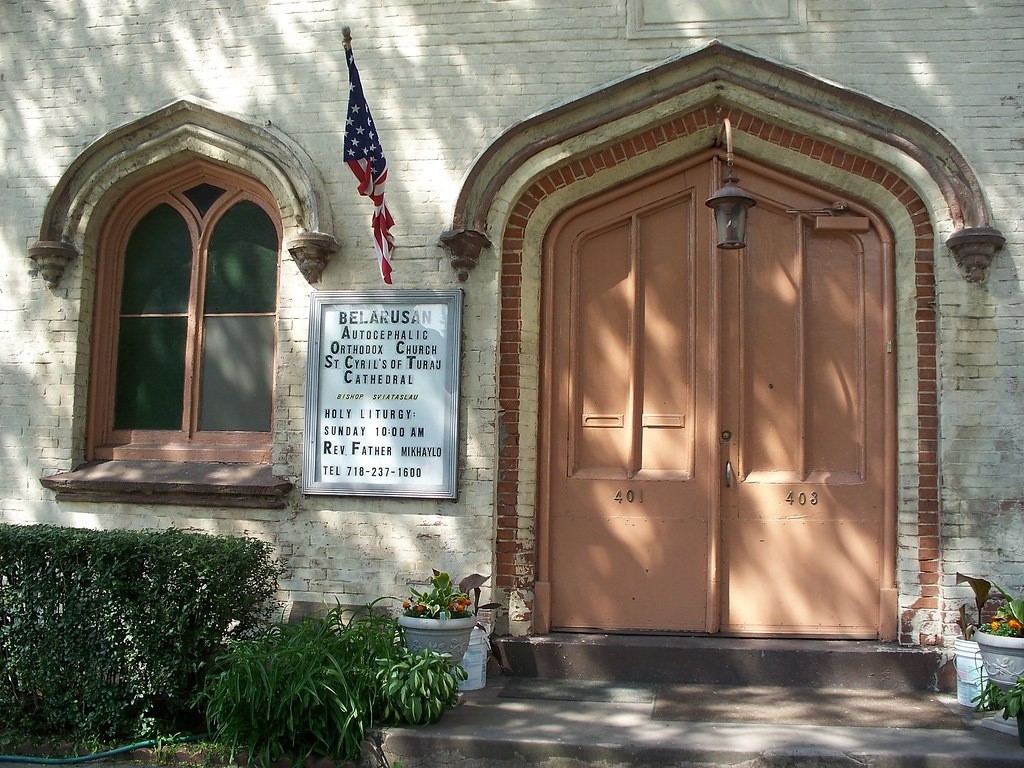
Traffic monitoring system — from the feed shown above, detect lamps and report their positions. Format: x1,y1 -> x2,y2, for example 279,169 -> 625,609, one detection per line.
705,118 -> 758,250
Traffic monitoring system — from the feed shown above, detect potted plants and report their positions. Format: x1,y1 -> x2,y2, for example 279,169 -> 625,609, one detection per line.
374,646 -> 468,727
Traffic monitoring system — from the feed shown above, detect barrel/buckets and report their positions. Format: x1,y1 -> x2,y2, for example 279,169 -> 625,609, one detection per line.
450,622 -> 492,690
953,636 -> 988,709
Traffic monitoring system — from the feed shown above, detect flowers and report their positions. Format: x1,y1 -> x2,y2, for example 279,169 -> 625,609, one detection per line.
393,570 -> 477,618
979,579 -> 1024,637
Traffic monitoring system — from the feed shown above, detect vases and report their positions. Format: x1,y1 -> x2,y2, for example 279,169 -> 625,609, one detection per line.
973,630 -> 1024,736
397,613 -> 477,706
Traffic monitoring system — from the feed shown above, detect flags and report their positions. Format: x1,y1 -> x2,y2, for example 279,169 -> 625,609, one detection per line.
342,50 -> 396,284
